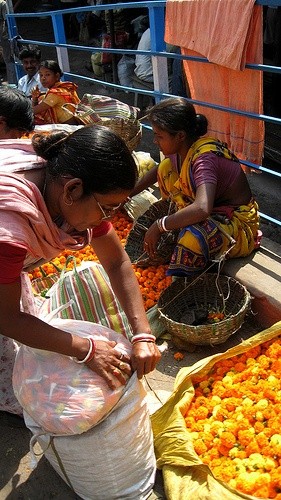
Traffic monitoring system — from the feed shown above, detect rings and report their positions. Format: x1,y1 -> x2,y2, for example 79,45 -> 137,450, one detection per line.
119,353 -> 124,360
145,242 -> 148,246
118,362 -> 128,370
112,367 -> 121,376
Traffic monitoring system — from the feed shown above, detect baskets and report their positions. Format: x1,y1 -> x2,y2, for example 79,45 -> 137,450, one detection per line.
157,274 -> 252,345
125,199 -> 177,268
84,118 -> 144,153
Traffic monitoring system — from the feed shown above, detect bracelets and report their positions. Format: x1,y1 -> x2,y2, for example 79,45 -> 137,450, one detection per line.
73,337 -> 92,364
84,338 -> 96,363
31,98 -> 39,102
127,197 -> 131,202
157,216 -> 170,233
131,333 -> 156,344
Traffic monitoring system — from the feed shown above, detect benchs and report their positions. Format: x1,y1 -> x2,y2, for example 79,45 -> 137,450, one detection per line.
222,248 -> 281,330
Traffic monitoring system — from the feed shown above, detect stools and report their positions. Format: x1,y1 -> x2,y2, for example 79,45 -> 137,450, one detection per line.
127,73 -> 153,107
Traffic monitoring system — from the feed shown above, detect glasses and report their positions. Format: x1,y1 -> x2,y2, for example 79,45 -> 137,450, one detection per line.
90,192 -> 116,222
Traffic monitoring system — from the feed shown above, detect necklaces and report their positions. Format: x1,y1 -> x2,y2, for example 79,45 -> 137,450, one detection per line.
41,179 -> 47,195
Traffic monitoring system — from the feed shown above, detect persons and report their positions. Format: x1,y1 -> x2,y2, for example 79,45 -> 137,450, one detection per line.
0,0 -> 18,87
17,45 -> 47,96
134,28 -> 173,110
0,84 -> 35,140
29,60 -> 85,126
0,124 -> 162,417
105,99 -> 262,275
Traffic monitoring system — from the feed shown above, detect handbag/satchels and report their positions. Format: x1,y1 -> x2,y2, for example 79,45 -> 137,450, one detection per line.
41,256 -> 135,341
12,299 -> 134,435
